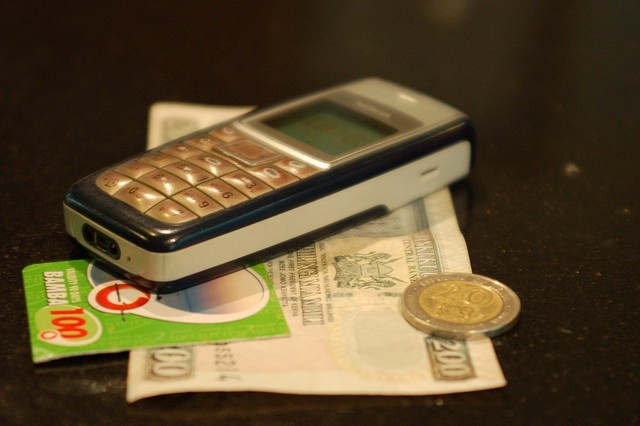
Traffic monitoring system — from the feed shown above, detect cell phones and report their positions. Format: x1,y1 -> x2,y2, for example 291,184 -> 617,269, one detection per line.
60,75 -> 477,295
